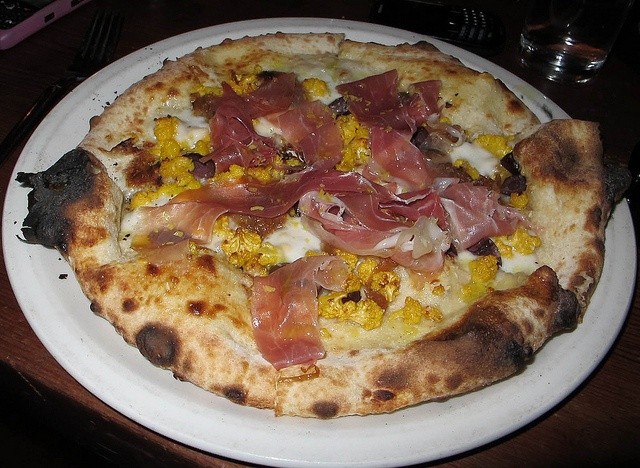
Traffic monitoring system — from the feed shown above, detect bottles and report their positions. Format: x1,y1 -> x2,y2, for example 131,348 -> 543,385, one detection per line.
519,0 -> 634,87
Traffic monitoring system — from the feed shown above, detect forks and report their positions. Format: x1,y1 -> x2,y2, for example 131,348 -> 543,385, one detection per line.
2,8 -> 124,164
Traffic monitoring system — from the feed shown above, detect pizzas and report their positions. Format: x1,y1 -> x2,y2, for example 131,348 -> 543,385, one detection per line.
14,32 -> 633,419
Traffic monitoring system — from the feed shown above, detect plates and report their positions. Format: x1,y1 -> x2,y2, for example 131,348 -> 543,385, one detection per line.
2,18 -> 638,467
